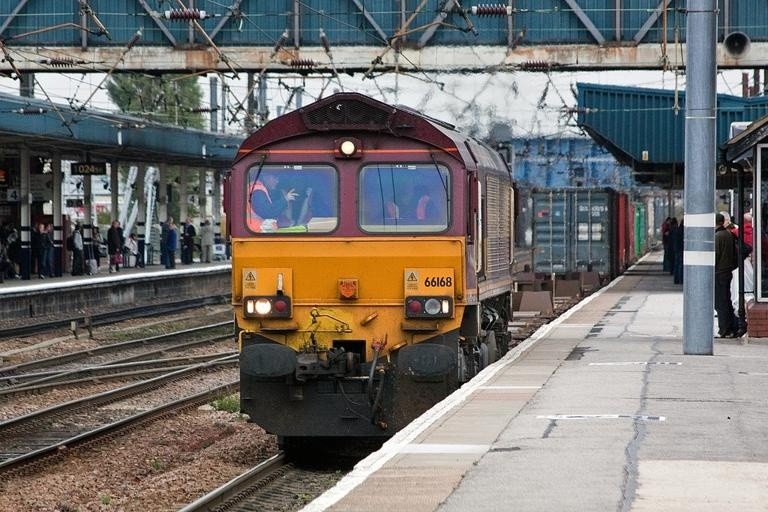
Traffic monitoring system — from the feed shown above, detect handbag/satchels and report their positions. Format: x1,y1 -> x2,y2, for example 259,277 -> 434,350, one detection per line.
735,242 -> 752,267
112,255 -> 123,264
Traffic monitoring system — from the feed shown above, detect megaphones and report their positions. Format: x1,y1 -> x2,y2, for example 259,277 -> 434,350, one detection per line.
722,31 -> 752,60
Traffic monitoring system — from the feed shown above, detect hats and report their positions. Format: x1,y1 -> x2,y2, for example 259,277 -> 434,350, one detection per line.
716,214 -> 724,224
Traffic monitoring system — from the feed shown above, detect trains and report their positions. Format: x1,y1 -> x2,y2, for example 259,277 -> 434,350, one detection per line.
220,93 -> 666,471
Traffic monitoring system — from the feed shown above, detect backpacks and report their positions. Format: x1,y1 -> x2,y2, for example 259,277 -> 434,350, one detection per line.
66,232 -> 81,250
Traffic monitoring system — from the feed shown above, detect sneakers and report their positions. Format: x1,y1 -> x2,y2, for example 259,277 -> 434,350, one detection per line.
714,332 -> 735,339
40,274 -> 45,278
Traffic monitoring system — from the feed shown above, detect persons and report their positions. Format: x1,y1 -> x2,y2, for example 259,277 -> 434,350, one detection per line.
386,174 -> 442,226
246,173 -> 301,234
712,210 -> 752,340
271,171 -> 333,231
661,215 -> 684,286
0,216 -> 228,282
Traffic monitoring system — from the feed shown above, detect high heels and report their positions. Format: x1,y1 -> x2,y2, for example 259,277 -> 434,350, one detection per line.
15,274 -> 21,279
